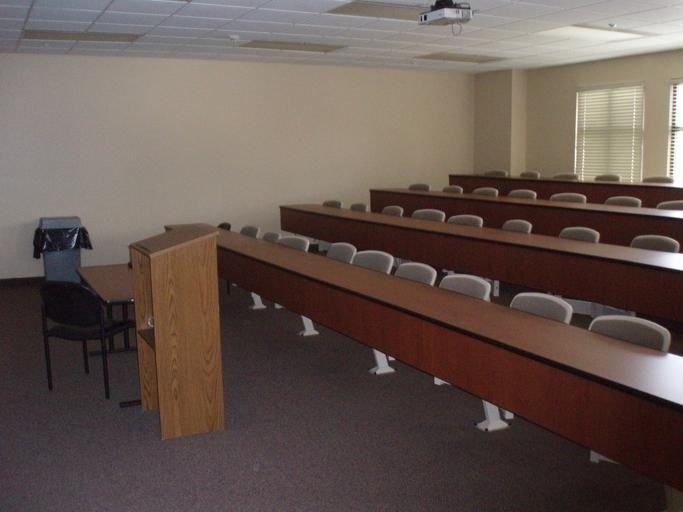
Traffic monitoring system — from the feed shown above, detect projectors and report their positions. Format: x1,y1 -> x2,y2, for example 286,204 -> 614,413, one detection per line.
417,7 -> 472,26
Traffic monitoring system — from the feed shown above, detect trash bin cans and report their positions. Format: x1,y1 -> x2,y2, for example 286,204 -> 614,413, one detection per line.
38,216 -> 82,283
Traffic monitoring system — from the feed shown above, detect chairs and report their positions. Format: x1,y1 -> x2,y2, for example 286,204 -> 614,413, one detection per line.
215,170 -> 682,468
38,278 -> 136,402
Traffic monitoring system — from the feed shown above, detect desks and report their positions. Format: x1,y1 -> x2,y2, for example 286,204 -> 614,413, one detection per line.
163,223 -> 682,490
72,264 -> 140,408
